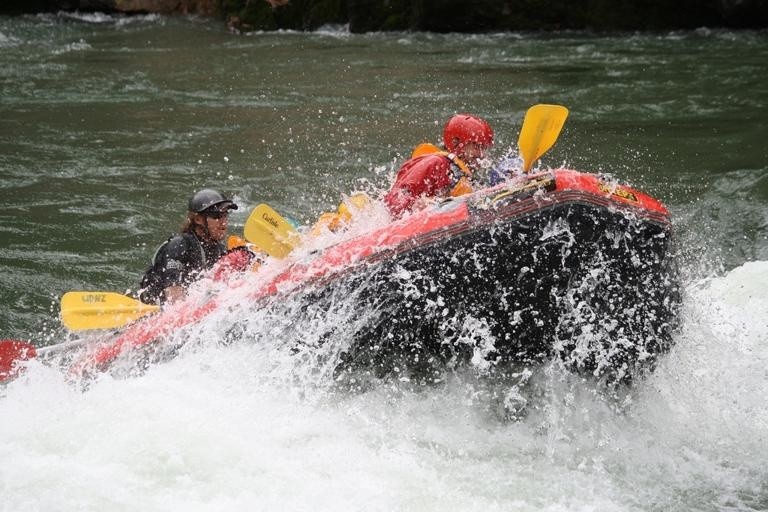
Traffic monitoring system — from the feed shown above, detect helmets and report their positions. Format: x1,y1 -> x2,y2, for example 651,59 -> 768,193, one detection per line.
189,188 -> 233,212
445,115 -> 493,159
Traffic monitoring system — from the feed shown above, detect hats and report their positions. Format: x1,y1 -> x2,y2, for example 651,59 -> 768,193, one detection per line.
204,202 -> 238,214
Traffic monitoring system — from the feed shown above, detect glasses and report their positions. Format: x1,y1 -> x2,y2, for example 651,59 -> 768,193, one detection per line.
208,213 -> 229,218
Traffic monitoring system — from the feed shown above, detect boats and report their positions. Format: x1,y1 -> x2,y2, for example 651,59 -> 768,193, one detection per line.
0,167 -> 681,398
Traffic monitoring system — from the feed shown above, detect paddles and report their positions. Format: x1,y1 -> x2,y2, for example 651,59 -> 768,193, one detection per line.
245,204 -> 301,262
59,290 -> 165,329
0,335 -> 114,385
518,104 -> 569,175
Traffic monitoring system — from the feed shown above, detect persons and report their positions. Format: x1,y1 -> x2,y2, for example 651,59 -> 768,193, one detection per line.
380,110 -> 494,224
137,188 -> 240,312
